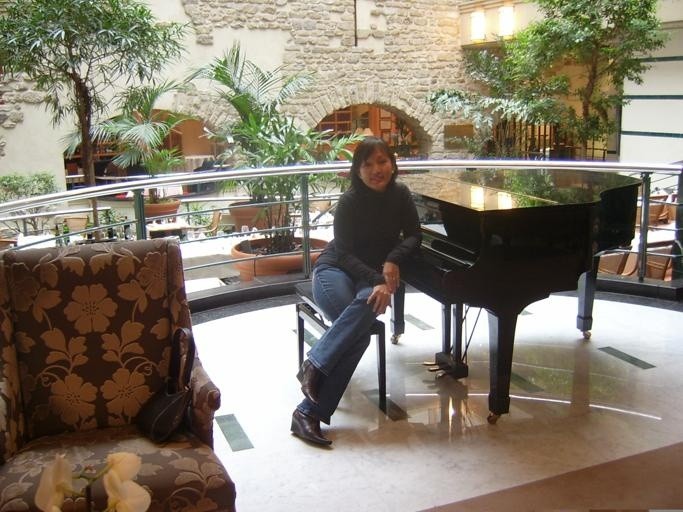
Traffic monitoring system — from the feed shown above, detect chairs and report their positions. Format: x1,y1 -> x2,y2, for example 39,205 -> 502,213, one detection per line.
597,186 -> 680,281
0,235 -> 236,512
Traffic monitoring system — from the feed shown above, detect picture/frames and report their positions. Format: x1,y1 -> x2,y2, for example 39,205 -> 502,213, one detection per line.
379,107 -> 392,145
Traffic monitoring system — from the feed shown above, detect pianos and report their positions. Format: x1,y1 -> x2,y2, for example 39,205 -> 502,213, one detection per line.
391,168 -> 644,423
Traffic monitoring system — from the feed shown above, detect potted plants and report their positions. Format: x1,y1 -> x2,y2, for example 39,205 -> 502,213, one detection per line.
233,132 -> 366,279
190,47 -> 313,227
62,79 -> 181,238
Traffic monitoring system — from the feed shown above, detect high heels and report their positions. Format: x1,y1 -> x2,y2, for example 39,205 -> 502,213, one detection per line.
297,360 -> 322,407
291,409 -> 332,445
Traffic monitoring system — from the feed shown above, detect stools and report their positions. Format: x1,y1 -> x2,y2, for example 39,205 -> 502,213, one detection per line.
295,283 -> 386,414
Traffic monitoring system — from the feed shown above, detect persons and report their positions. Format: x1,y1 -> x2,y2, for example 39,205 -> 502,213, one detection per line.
289,135 -> 422,445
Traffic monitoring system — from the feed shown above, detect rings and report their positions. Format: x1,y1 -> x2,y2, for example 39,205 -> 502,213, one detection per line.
392,277 -> 395,280
